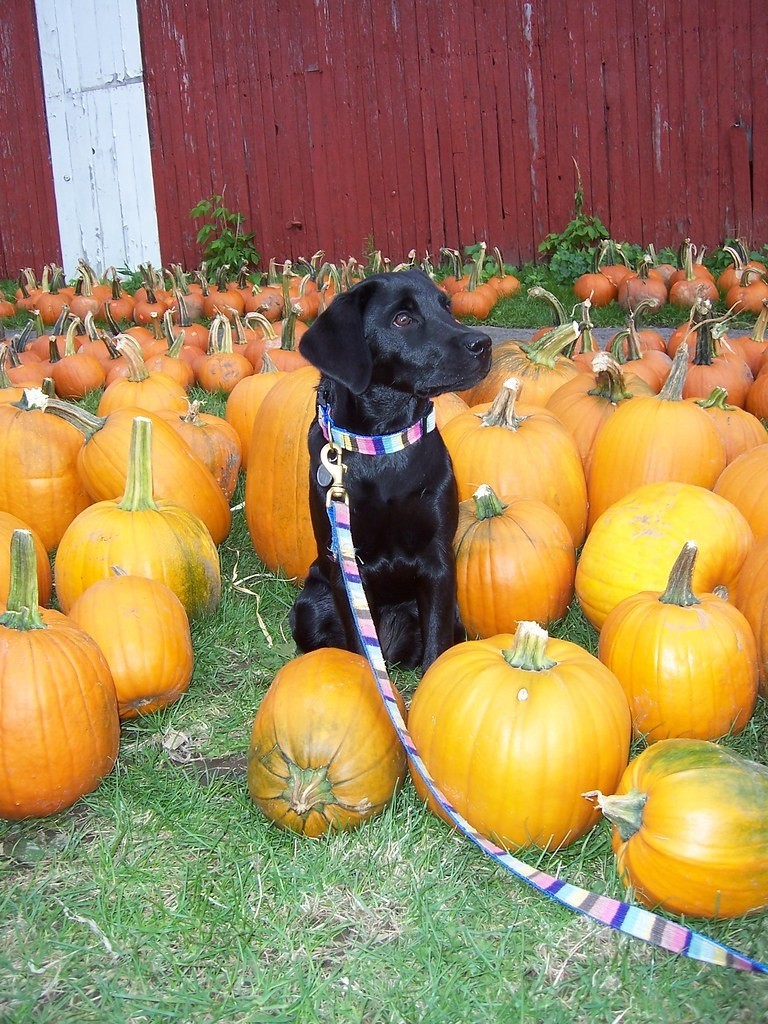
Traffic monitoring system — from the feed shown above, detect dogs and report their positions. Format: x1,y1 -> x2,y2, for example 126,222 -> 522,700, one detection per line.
287,269 -> 492,684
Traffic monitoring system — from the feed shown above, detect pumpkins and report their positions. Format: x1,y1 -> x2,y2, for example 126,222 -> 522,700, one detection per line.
0,241 -> 768,921
